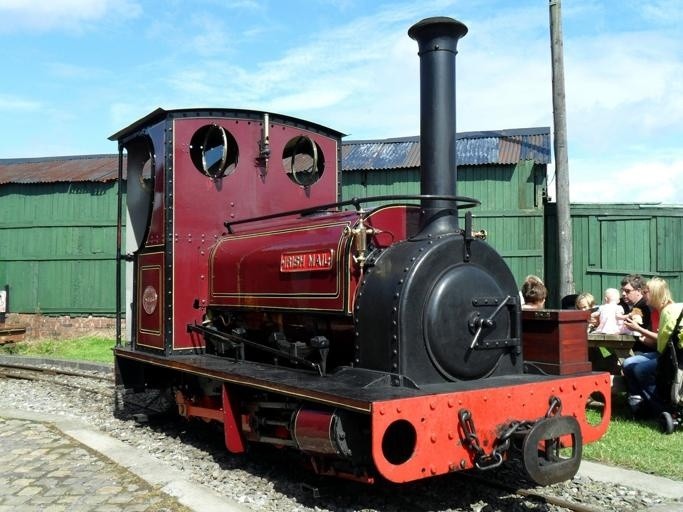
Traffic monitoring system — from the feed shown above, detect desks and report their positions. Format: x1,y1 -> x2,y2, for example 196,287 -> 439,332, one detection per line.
586,333 -> 635,374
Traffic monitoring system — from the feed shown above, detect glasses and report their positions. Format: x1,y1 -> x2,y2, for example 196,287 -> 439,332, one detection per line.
621,287 -> 639,293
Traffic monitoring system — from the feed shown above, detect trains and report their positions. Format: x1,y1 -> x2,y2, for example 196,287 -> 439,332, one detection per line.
92,14 -> 612,504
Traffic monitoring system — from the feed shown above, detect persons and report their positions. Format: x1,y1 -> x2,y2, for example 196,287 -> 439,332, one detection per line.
518,272 -> 682,417
